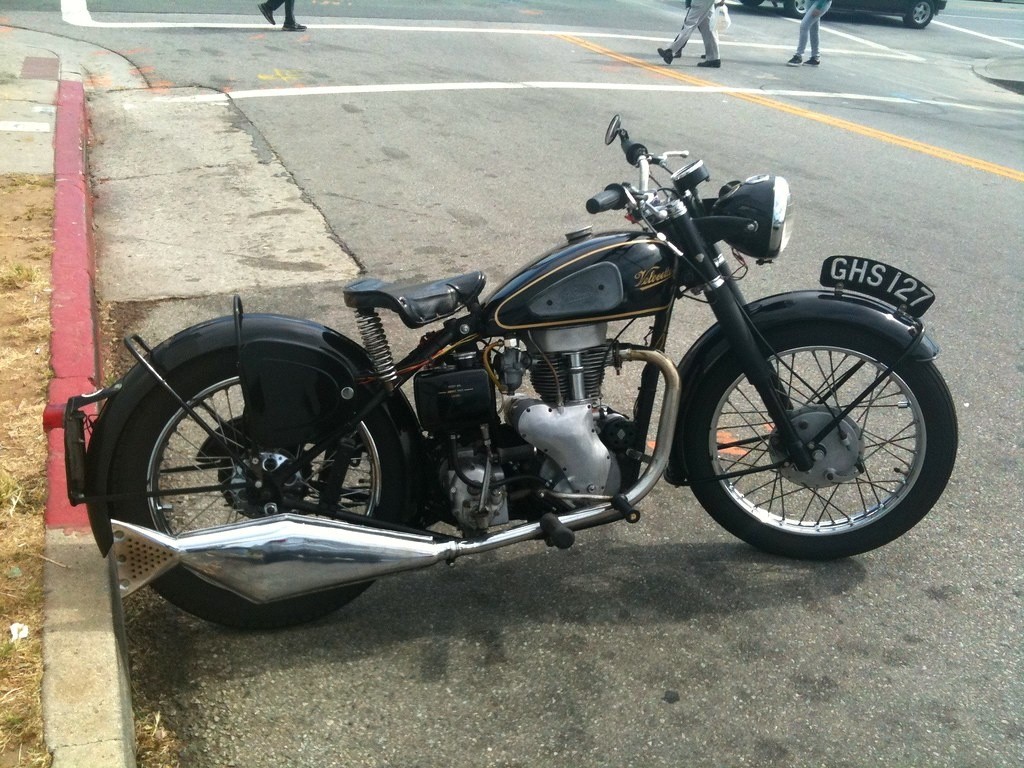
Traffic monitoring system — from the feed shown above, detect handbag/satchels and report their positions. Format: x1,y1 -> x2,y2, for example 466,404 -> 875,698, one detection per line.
715,4 -> 731,33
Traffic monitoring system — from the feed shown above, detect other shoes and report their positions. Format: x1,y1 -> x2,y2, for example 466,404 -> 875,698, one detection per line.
701,55 -> 706,58
658,48 -> 673,64
675,50 -> 682,57
697,59 -> 720,67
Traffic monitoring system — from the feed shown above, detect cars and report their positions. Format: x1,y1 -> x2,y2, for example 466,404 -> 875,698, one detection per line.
739,0 -> 947,29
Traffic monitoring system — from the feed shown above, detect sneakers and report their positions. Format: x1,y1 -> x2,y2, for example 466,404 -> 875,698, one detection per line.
788,55 -> 802,65
282,21 -> 306,31
258,3 -> 275,25
803,57 -> 820,66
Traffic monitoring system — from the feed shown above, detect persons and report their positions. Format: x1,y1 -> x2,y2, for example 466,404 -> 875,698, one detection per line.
258,0 -> 307,31
657,0 -> 726,69
787,0 -> 832,67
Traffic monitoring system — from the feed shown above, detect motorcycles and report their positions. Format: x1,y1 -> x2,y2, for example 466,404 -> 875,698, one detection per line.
64,115 -> 958,630
